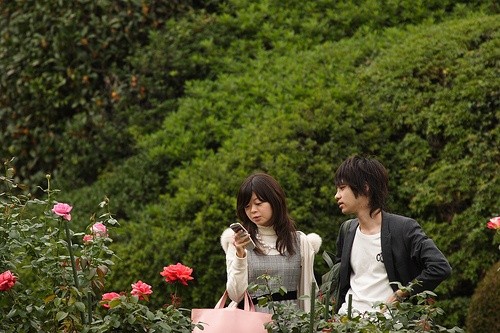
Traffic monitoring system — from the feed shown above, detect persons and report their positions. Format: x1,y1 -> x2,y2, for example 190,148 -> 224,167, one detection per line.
220,172 -> 320,333
325,153 -> 452,324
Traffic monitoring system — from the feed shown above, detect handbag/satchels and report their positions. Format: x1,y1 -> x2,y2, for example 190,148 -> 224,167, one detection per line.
321,251 -> 341,308
189,287 -> 273,333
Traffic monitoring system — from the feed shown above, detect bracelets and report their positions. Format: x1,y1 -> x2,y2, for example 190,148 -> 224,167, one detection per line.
394,292 -> 407,302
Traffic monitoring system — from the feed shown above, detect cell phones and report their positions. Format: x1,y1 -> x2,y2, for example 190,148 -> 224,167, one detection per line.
230,223 -> 256,251
